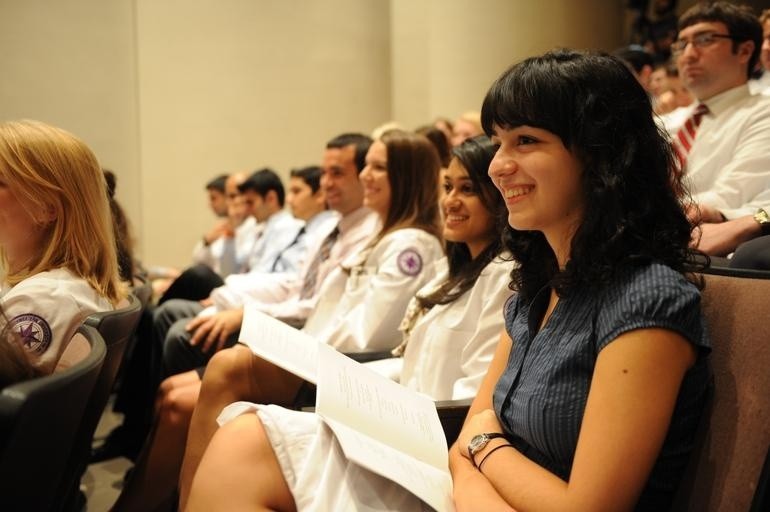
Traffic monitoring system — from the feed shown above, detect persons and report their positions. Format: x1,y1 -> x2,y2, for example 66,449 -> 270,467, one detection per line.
617,1 -> 770,271
87,45 -> 717,509
3,117 -> 149,389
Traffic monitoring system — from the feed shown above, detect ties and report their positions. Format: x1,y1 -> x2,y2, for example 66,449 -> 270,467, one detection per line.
271,226 -> 305,276
299,227 -> 339,301
667,103 -> 709,182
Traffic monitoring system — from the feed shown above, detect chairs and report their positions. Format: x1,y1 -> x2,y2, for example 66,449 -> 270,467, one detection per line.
0,323 -> 108,512
126,273 -> 154,357
86,287 -> 142,439
682,262 -> 769,510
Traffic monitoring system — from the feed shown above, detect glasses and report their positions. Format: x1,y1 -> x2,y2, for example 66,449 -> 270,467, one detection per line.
670,32 -> 729,56
